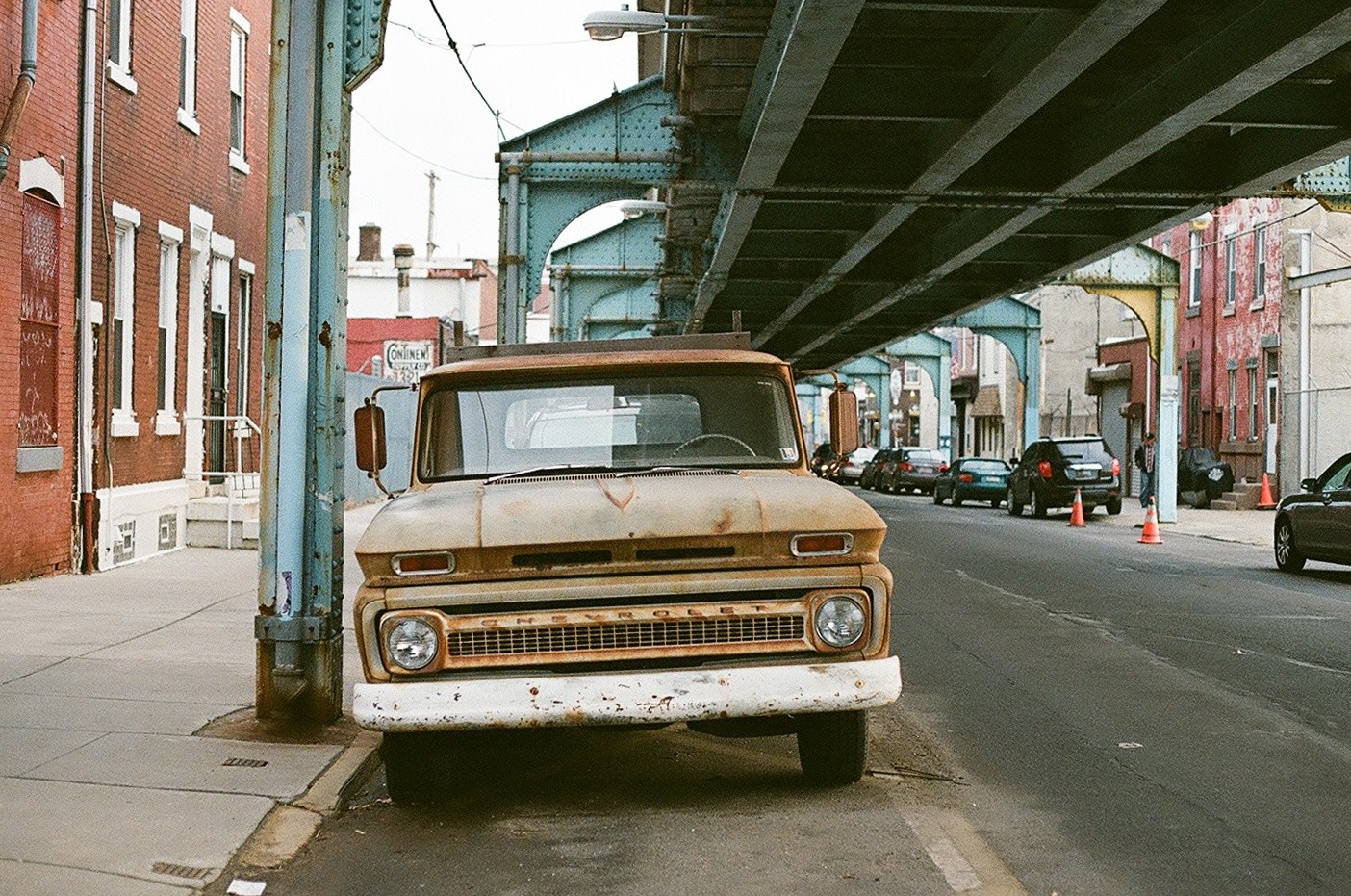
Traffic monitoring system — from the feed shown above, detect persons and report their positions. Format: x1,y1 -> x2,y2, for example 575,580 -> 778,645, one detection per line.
1134,434 -> 1157,508
814,440 -> 836,477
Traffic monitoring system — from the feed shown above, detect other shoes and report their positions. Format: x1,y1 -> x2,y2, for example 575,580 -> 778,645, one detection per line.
1142,503 -> 1148,508
1149,500 -> 1154,505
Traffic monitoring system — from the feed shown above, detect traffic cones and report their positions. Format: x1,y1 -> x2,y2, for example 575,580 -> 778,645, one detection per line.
1256,471 -> 1280,507
1067,485 -> 1089,528
1137,493 -> 1164,546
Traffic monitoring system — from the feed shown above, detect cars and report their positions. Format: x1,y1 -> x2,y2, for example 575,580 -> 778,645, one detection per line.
882,445 -> 948,493
346,333 -> 906,785
1271,451 -> 1351,577
837,445 -> 889,489
933,457 -> 1015,508
1006,434 -> 1121,517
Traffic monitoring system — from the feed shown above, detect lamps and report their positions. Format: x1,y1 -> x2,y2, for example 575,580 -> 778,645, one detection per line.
584,10 -> 716,40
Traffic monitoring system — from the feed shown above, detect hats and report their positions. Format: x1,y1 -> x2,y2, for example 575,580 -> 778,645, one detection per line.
1145,432 -> 1154,440
